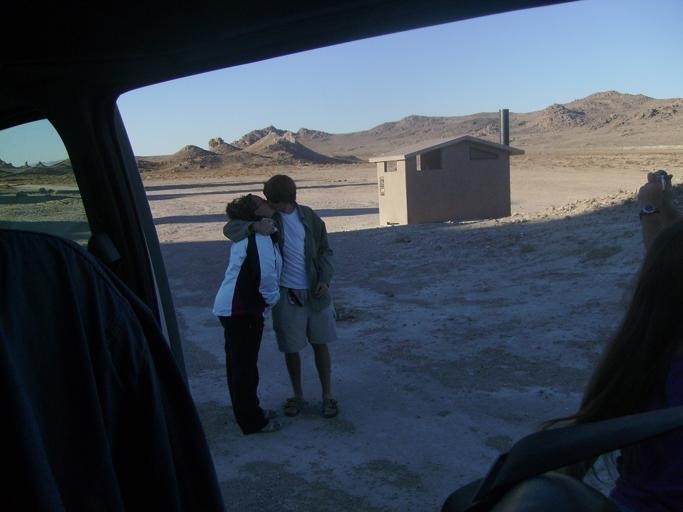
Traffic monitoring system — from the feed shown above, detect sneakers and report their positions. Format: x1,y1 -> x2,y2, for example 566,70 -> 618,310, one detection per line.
260,420 -> 280,431
322,398 -> 337,417
286,397 -> 305,415
260,409 -> 281,418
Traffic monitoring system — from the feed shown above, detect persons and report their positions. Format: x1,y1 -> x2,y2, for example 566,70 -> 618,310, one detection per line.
222,175 -> 340,418
507,218 -> 683,512
212,193 -> 287,435
636,167 -> 682,252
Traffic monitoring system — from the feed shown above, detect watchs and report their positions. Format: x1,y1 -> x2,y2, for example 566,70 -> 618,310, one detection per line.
639,202 -> 659,219
